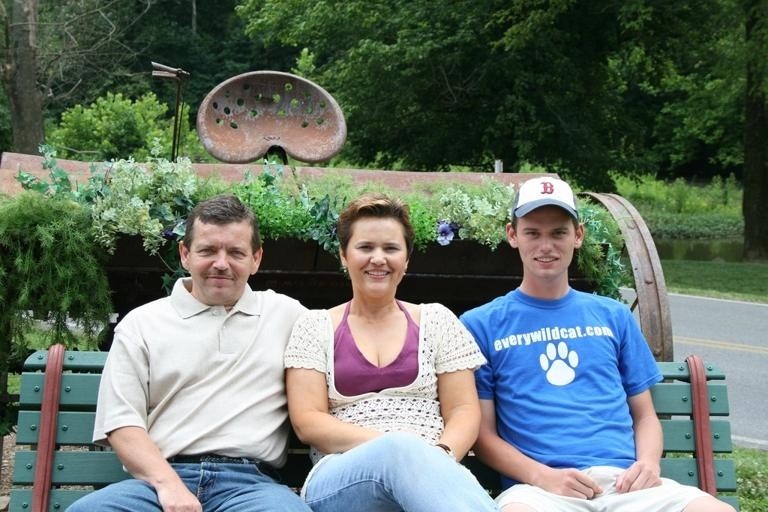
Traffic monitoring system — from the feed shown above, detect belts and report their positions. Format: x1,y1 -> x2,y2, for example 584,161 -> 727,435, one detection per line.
169,454 -> 256,465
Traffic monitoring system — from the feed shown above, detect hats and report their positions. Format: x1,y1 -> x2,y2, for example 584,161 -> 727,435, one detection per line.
511,175 -> 580,224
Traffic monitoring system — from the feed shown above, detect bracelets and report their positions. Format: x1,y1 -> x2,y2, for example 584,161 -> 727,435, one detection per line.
437,440 -> 457,459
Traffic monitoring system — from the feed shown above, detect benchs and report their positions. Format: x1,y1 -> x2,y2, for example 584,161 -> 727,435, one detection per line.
7,346 -> 741,512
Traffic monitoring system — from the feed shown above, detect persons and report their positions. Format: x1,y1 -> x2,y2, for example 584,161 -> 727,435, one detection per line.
283,189 -> 506,511
65,193 -> 320,512
457,173 -> 740,512
